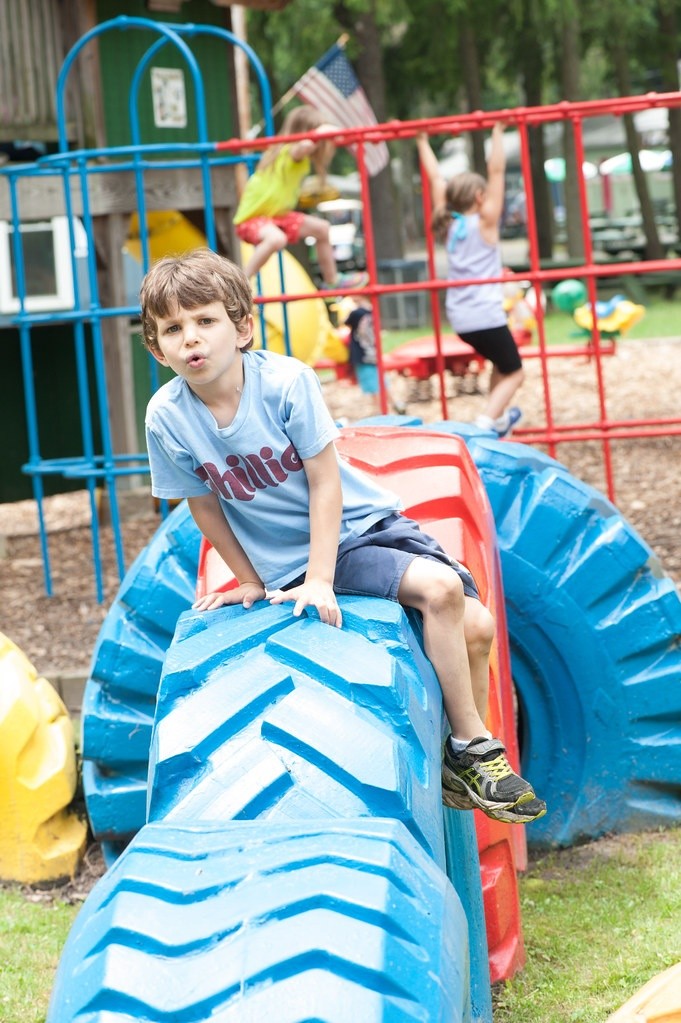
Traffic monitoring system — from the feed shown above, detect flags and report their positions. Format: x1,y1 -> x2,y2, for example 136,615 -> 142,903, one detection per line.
293,42 -> 391,177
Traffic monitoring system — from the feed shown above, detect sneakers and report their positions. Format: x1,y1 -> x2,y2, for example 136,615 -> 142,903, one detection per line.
322,269 -> 369,290
444,728 -> 533,800
445,785 -> 546,824
493,408 -> 522,438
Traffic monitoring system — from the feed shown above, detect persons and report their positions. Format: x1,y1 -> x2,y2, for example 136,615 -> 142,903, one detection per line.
415,120 -> 522,440
231,105 -> 370,293
137,246 -> 547,824
343,308 -> 380,416
